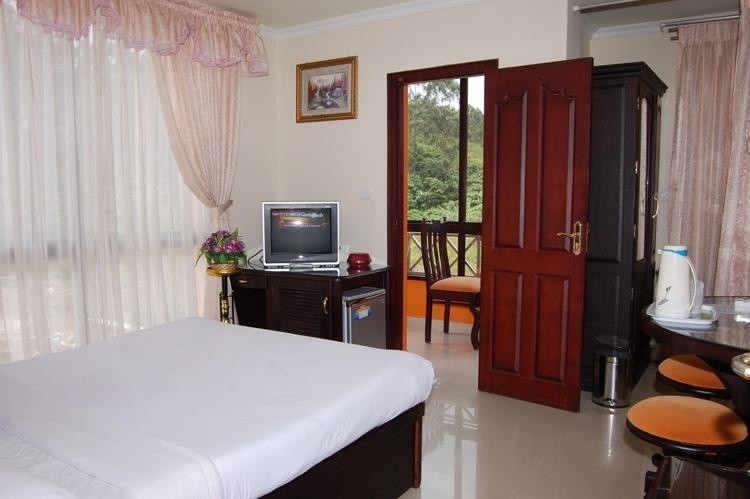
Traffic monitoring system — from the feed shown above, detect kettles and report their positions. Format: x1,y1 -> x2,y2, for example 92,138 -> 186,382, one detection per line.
656,245 -> 696,321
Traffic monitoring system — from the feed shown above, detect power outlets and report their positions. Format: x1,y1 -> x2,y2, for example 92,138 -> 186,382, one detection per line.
340,244 -> 349,255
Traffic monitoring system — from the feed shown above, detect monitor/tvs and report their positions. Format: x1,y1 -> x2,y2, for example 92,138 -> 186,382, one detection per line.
262,199 -> 340,275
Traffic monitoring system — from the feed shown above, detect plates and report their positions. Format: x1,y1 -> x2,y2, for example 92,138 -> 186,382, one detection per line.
646,301 -> 719,328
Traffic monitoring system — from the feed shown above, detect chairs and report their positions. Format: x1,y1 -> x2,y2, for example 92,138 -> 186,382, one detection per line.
420,216 -> 481,351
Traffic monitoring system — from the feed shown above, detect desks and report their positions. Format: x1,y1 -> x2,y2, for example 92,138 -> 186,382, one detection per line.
638,295 -> 750,365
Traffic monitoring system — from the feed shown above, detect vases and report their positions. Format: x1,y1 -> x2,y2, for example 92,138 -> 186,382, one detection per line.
211,262 -> 237,272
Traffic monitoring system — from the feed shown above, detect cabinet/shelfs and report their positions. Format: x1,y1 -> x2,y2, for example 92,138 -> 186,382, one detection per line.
580,60 -> 669,392
233,272 -> 388,350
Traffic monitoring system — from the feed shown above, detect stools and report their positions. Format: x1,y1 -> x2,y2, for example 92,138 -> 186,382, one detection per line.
626,393 -> 750,499
656,354 -> 730,398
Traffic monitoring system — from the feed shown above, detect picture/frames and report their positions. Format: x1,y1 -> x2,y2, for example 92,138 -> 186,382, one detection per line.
296,55 -> 358,123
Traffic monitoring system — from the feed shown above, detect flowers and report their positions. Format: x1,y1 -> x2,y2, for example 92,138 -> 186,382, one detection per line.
193,228 -> 248,270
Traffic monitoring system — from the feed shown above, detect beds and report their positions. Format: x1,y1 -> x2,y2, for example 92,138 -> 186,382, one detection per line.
1,317 -> 435,499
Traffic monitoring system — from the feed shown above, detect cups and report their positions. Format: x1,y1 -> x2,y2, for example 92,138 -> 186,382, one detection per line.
690,279 -> 706,316
733,300 -> 750,326
